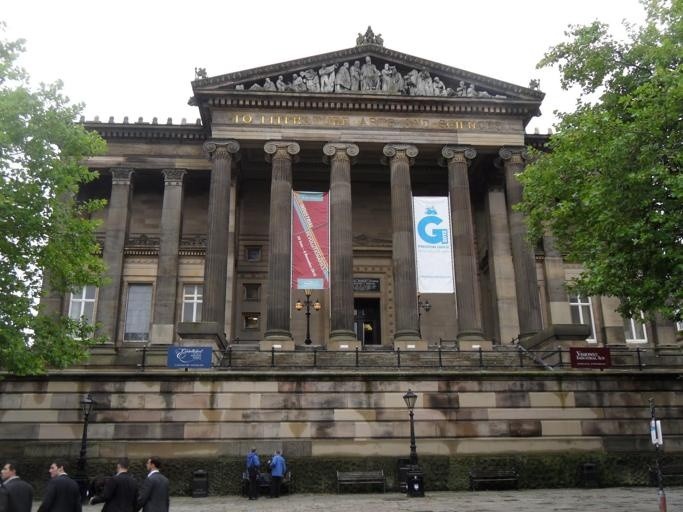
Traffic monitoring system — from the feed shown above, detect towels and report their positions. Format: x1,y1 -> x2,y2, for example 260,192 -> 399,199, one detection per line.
581,463 -> 601,488
396,458 -> 412,493
191,469 -> 208,498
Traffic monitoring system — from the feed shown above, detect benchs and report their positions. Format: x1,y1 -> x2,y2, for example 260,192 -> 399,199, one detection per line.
363,323 -> 372,331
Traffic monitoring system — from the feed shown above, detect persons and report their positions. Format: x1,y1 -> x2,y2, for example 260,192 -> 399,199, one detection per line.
263,56 -> 478,97
137,455 -> 170,512
246,447 -> 260,500
37,458 -> 82,512
89,456 -> 139,512
0,460 -> 33,512
268,450 -> 286,499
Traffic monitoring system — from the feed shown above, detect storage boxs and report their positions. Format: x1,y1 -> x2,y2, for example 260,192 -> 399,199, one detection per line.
467,465 -> 520,492
336,469 -> 387,494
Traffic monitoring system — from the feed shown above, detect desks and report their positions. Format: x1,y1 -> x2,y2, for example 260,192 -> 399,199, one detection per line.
400,388 -> 426,498
70,392 -> 96,501
292,286 -> 323,344
415,292 -> 431,339
